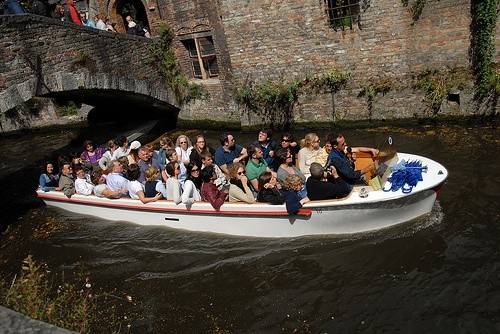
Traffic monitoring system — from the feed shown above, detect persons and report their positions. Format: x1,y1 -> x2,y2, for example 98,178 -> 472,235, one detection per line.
126,163 -> 162,204
182,163 -> 202,204
327,132 -> 376,186
228,162 -> 257,204
306,162 -> 351,201
59,163 -> 76,196
200,165 -> 228,210
144,166 -> 167,200
324,139 -> 333,153
284,174 -> 310,216
72,127 -> 300,199
165,162 -> 184,203
276,149 -> 306,184
53,0 -> 118,33
298,132 -> 329,178
40,161 -> 60,192
3,0 -> 53,18
126,16 -> 151,38
256,171 -> 285,206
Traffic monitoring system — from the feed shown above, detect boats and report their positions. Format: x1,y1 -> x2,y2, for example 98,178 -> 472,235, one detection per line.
34,152 -> 449,237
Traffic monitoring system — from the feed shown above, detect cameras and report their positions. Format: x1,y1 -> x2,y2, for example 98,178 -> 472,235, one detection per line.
323,166 -> 330,172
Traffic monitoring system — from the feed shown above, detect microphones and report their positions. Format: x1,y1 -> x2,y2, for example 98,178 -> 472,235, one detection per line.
347,146 -> 352,155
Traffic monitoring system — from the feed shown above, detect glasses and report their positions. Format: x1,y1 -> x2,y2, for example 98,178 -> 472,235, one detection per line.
191,168 -> 200,172
313,138 -> 320,143
236,170 -> 246,176
174,165 -> 180,170
286,154 -> 291,158
281,138 -> 290,142
180,142 -> 187,144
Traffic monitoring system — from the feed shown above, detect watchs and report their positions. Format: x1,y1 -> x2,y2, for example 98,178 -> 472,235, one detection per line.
118,190 -> 121,194
